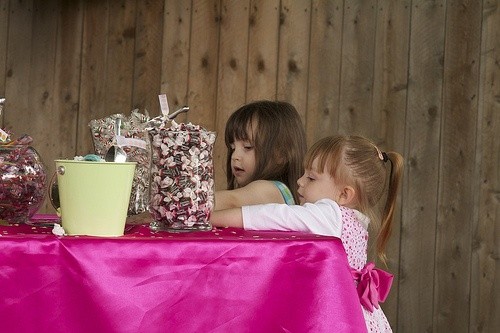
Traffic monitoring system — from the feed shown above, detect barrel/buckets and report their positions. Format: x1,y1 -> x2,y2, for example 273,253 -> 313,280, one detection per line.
52,159 -> 138,238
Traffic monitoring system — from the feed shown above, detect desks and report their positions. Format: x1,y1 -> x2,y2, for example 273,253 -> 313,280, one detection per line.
0,223 -> 368,333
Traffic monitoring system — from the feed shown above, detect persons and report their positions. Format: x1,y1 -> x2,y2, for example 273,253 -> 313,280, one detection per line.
213,100 -> 309,211
207,134 -> 405,333
